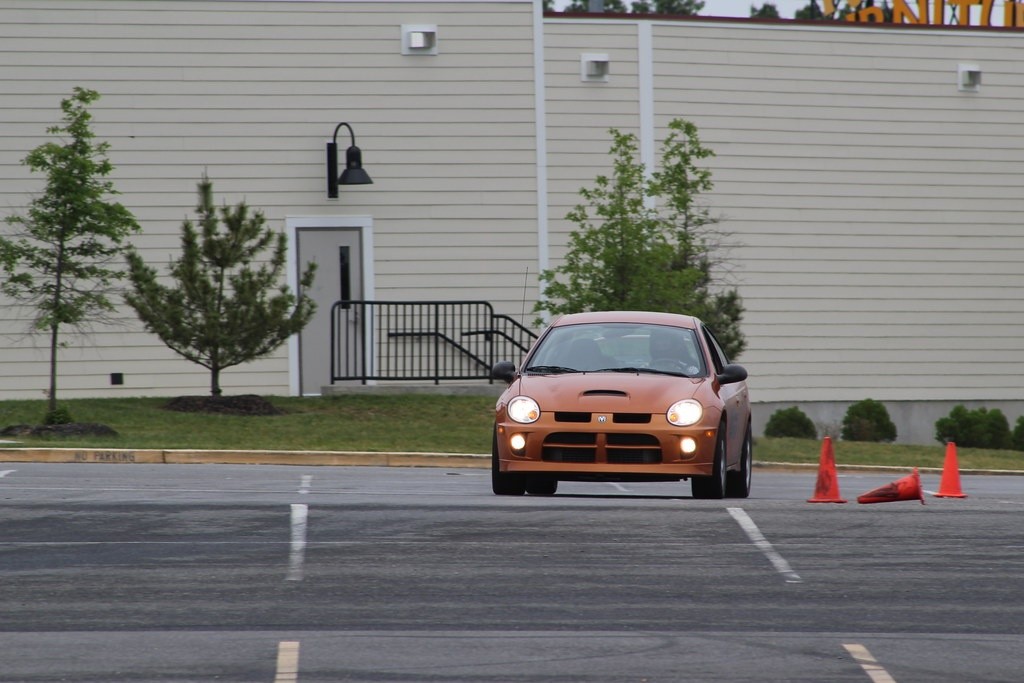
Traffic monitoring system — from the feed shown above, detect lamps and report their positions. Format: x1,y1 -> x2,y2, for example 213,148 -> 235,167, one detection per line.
580,52 -> 610,82
958,63 -> 982,92
325,123 -> 372,198
400,23 -> 438,56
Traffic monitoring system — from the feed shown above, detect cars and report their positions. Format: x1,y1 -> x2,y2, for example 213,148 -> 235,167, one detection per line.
490,311 -> 752,497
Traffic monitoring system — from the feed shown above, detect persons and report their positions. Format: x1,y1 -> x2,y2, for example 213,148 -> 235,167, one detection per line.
640,331 -> 700,378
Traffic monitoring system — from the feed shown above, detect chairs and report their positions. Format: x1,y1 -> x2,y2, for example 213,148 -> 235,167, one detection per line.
562,339 -> 618,370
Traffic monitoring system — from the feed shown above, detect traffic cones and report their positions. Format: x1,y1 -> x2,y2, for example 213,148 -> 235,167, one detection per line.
807,436 -> 846,503
931,438 -> 969,498
856,466 -> 926,507
